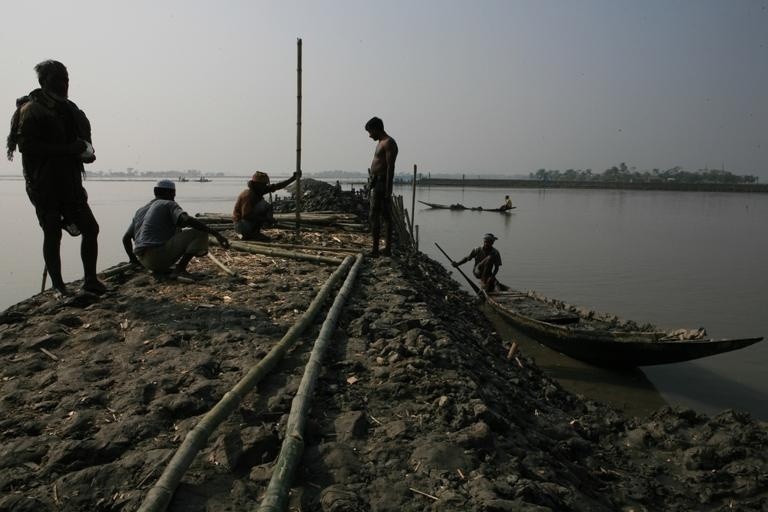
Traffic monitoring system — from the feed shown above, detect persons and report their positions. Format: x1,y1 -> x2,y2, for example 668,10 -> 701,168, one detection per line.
121,178 -> 231,283
364,115 -> 399,258
231,169 -> 303,242
450,232 -> 502,296
496,194 -> 513,210
6,59 -> 118,306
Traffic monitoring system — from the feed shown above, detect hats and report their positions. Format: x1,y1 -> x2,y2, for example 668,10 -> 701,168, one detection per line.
252,171 -> 270,184
38,59 -> 69,80
153,178 -> 177,190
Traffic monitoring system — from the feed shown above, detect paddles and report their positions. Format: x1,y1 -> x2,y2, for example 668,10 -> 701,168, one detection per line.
434,242 -> 486,300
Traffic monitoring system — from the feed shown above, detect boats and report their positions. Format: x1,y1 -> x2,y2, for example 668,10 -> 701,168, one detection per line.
481,276 -> 765,369
192,180 -> 213,182
417,199 -> 517,211
170,180 -> 189,182
